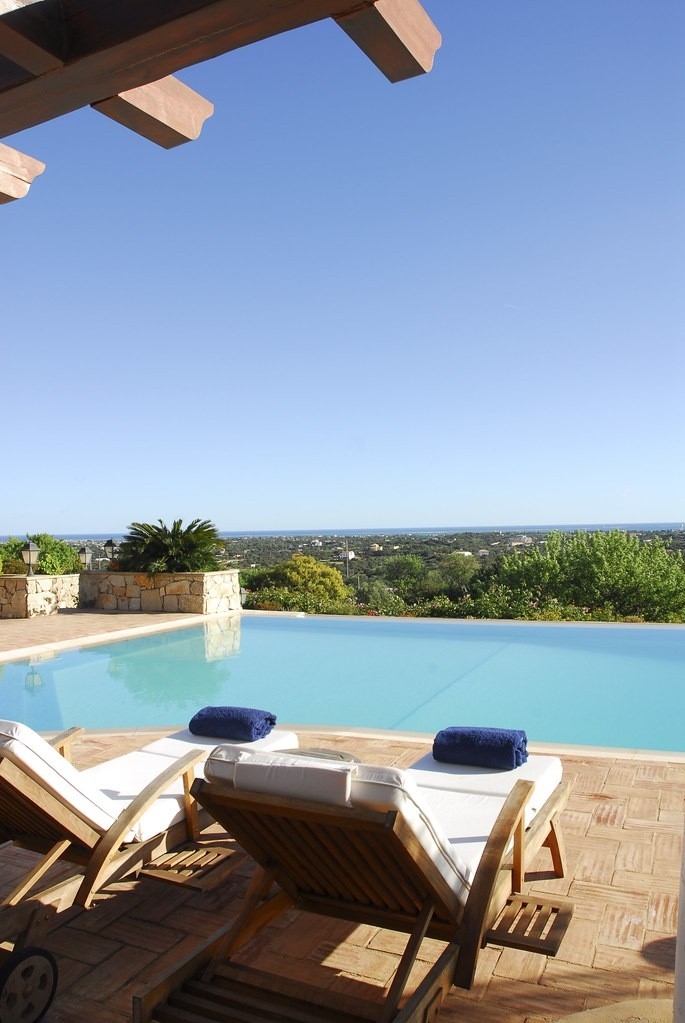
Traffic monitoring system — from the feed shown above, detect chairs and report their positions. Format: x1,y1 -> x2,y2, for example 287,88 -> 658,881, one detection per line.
127,723 -> 575,1023
0,706 -> 300,1022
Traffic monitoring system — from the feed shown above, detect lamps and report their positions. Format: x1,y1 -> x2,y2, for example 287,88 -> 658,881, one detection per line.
77,547 -> 94,564
18,542 -> 44,577
102,539 -> 121,559
